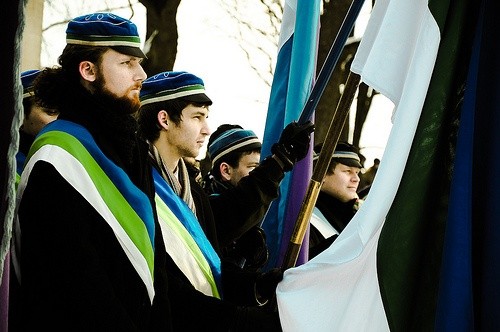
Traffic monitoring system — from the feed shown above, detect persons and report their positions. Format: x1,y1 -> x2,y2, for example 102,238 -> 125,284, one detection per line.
133,71 -> 287,311
301,141 -> 367,263
366,157 -> 381,182
194,120 -> 320,294
11,11 -> 283,332
14,68 -> 61,193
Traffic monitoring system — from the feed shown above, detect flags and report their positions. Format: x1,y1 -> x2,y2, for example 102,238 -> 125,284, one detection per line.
276,0 -> 499,332
261,1 -> 323,269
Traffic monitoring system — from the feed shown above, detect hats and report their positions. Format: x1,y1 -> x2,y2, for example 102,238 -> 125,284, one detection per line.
138,72 -> 212,107
20,69 -> 44,99
207,127 -> 261,165
314,140 -> 363,167
65,12 -> 149,59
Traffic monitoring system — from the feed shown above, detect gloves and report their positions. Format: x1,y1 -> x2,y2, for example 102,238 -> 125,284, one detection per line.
229,225 -> 268,273
259,266 -> 284,299
271,120 -> 316,172
